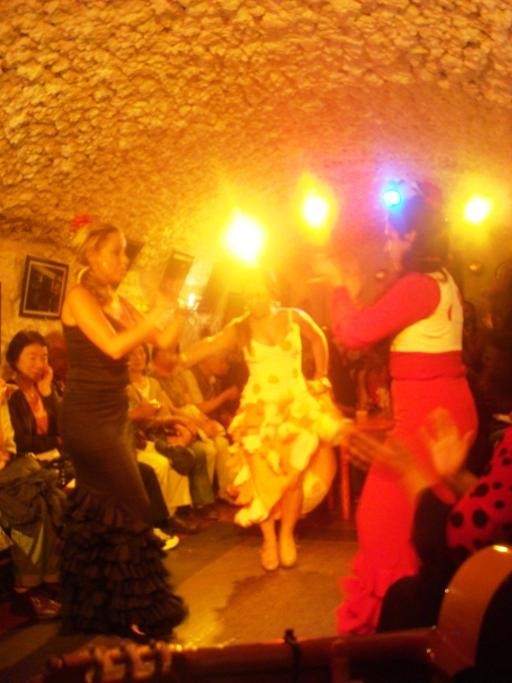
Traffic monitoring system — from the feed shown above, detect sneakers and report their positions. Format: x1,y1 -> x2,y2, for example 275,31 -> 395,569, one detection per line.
148,504 -> 222,552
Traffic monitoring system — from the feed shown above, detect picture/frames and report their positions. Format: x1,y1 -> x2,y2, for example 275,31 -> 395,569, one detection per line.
20,254 -> 68,319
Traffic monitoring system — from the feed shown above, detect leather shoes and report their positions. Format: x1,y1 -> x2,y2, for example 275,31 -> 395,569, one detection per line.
260,536 -> 297,572
8,592 -> 62,622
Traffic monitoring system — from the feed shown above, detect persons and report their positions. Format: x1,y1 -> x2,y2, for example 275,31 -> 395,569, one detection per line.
178,254 -> 352,571
289,191 -> 482,641
0,281 -> 511,681
51,219 -> 210,651
